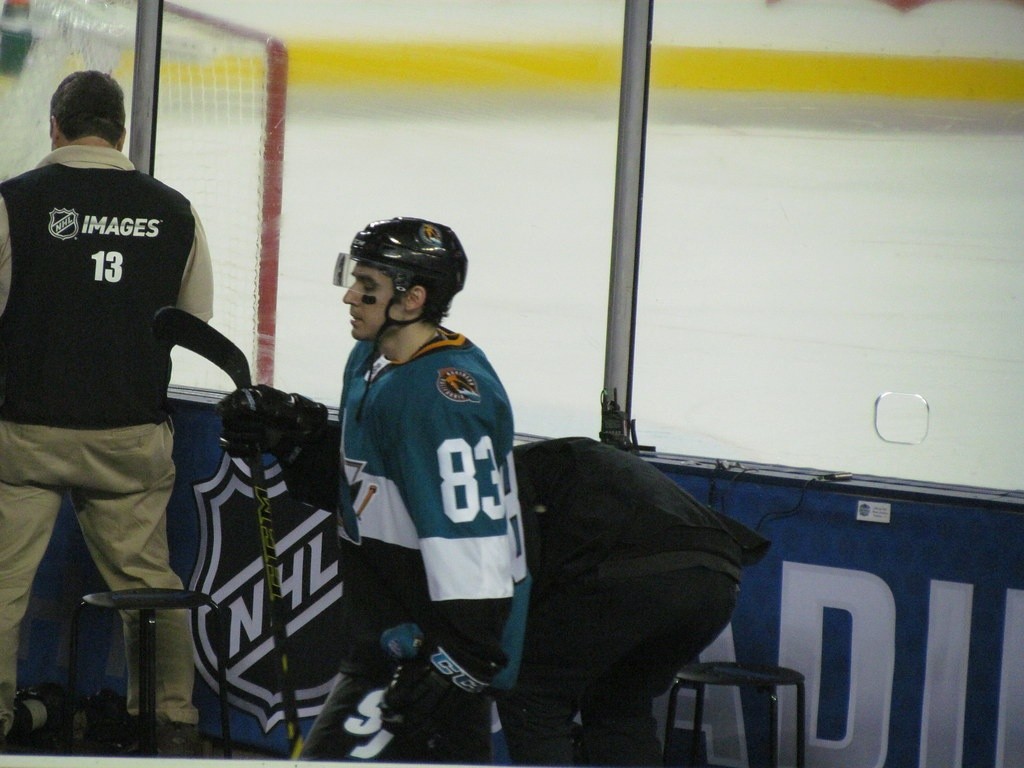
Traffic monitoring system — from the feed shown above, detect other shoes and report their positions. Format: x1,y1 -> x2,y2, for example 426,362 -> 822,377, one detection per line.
155,721 -> 198,755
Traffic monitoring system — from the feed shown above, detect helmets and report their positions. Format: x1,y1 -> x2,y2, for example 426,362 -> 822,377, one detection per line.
350,217 -> 467,307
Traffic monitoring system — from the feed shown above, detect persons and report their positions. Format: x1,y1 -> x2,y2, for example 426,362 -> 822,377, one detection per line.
215,216 -> 528,766
2,69 -> 219,760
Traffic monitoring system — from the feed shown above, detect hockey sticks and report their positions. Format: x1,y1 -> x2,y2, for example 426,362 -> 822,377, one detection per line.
150,303 -> 304,760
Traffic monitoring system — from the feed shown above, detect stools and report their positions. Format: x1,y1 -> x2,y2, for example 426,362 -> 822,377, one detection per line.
660,660 -> 807,768
68,587 -> 232,760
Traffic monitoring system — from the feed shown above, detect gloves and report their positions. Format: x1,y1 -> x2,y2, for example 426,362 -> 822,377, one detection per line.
379,617 -> 509,751
214,384 -> 329,457
